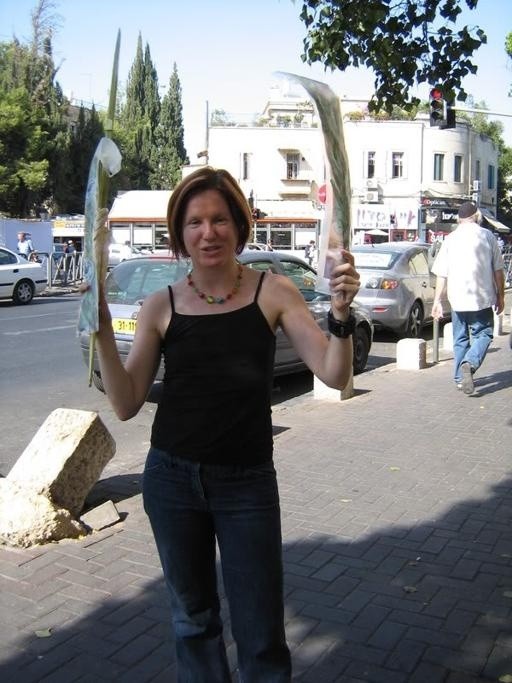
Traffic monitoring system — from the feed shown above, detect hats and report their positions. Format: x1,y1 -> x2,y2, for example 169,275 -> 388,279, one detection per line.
458,203 -> 477,218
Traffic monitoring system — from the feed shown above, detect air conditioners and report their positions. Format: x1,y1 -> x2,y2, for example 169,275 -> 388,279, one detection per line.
472,193 -> 480,202
364,191 -> 379,203
365,177 -> 378,189
473,179 -> 482,191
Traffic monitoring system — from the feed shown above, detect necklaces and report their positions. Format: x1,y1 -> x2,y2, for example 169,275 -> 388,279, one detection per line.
186,259 -> 243,304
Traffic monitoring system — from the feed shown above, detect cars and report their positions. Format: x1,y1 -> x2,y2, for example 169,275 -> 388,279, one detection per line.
0,246 -> 46,304
347,243 -> 451,339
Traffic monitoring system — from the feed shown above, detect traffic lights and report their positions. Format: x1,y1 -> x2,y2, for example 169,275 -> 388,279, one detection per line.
430,88 -> 456,130
251,208 -> 260,219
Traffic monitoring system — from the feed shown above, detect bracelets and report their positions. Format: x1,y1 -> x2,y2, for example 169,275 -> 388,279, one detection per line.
326,309 -> 358,338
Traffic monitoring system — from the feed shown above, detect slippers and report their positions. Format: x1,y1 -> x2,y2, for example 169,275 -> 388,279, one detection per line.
455,364 -> 475,394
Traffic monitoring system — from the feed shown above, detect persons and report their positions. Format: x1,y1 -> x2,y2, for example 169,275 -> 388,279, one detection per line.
497,235 -> 505,254
72,239 -> 80,258
16,231 -> 36,261
428,199 -> 505,394
63,238 -> 78,280
158,235 -> 169,245
79,162 -> 361,681
305,239 -> 316,265
265,239 -> 273,251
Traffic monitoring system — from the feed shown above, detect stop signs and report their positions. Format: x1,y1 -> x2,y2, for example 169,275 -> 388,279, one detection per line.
320,184 -> 326,202
